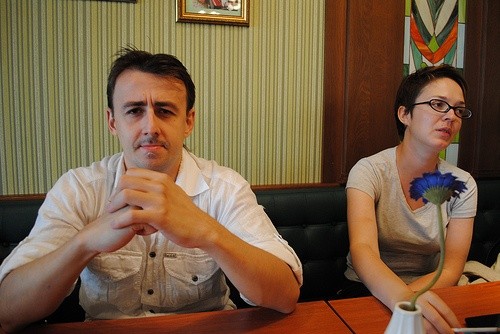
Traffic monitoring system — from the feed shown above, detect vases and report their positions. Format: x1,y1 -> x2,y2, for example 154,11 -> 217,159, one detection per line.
384,301 -> 426,334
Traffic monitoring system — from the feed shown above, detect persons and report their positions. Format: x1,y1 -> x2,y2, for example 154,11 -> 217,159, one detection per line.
0,43 -> 303,334
340,64 -> 478,334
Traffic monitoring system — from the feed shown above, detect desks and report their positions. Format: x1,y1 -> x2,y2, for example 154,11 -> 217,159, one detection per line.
20,281 -> 500,334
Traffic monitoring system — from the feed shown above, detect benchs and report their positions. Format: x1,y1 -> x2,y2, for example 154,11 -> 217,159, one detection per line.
0,169 -> 500,326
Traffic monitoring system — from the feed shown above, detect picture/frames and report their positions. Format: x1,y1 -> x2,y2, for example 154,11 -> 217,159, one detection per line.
175,0 -> 250,27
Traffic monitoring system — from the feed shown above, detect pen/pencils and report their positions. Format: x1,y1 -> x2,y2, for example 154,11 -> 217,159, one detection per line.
451,327 -> 500,333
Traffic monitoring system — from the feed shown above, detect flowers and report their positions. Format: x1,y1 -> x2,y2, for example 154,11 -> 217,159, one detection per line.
408,170 -> 468,311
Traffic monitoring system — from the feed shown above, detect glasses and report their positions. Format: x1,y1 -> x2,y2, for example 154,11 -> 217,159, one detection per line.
408,99 -> 473,120
